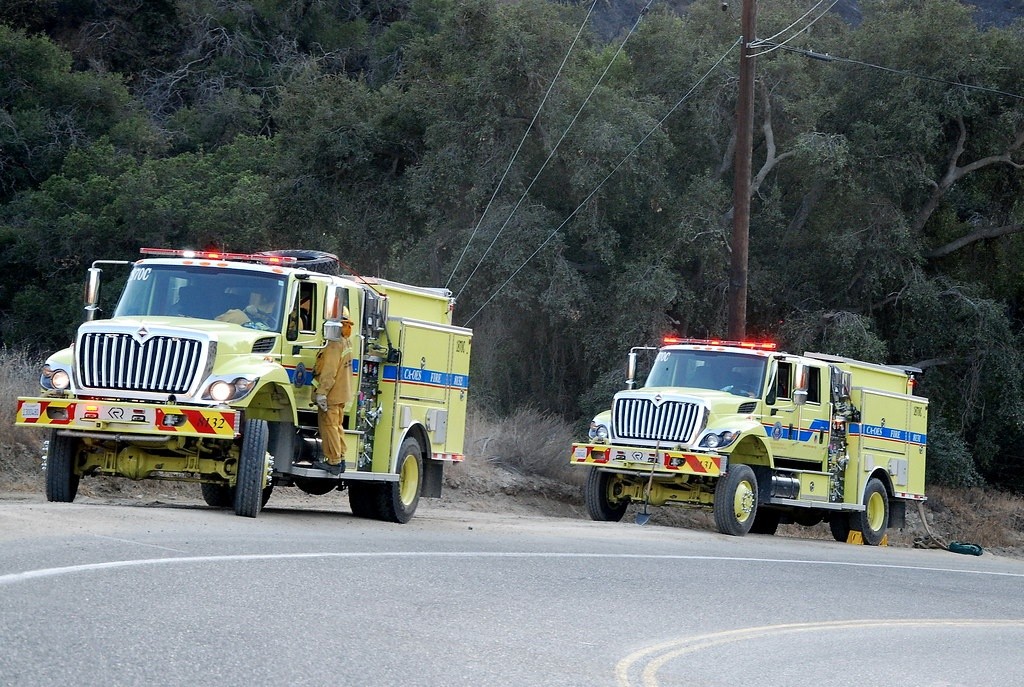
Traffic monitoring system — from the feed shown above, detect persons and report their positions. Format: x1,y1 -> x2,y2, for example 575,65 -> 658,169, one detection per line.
243,289 -> 303,331
311,306 -> 354,476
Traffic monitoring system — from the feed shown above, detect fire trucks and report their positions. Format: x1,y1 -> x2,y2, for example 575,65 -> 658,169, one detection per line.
15,247 -> 474,525
569,337 -> 930,547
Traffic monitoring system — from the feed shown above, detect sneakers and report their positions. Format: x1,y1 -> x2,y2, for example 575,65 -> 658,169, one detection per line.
313,461 -> 345,475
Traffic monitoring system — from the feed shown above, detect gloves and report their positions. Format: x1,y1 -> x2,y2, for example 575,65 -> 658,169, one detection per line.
315,393 -> 327,411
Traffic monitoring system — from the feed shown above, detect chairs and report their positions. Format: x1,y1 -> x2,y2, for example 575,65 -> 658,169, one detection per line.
170,285 -> 214,320
694,364 -> 732,391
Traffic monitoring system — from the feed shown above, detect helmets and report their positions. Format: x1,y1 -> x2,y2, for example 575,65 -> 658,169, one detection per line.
326,306 -> 349,320
